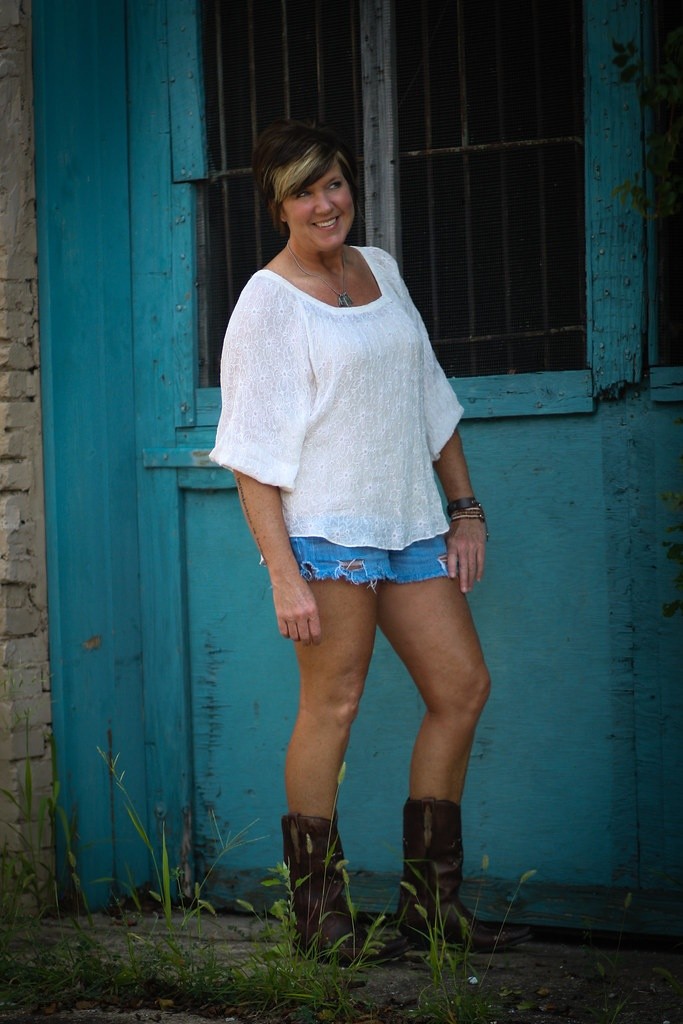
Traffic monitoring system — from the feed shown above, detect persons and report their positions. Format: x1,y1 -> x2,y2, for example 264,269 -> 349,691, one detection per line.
208,122 -> 525,970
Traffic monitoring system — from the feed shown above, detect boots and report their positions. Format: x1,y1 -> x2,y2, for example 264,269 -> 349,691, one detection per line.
399,800 -> 509,952
282,811 -> 359,965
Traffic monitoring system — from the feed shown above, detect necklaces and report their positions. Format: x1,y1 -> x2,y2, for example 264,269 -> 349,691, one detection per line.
287,240 -> 354,307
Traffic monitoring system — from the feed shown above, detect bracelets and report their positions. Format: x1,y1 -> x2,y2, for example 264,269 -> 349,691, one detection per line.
448,495 -> 485,521
